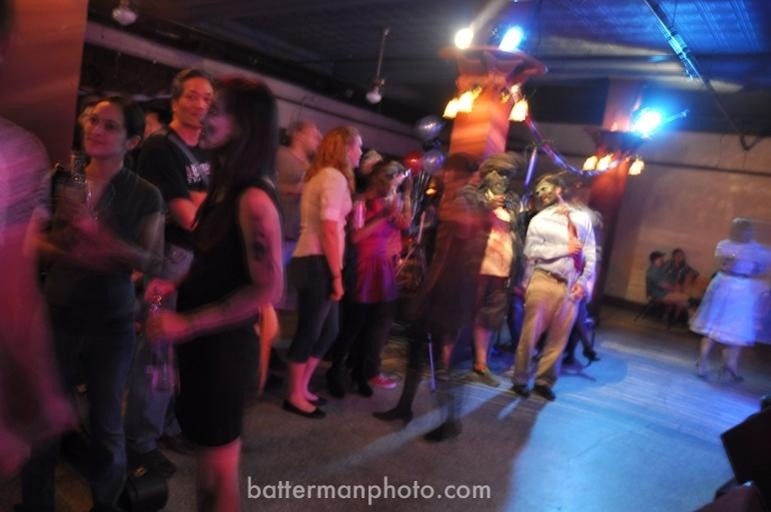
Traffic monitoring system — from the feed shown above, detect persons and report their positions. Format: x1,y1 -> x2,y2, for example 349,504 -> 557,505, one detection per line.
686,217 -> 771,384
697,395 -> 770,511
658,246 -> 701,322
1,62 -> 599,512
646,249 -> 690,324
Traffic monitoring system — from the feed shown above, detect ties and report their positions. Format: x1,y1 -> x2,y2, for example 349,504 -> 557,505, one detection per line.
556,209 -> 585,272
534,268 -> 568,284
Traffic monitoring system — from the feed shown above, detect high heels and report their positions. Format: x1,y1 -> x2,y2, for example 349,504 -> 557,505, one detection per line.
372,408 -> 414,428
423,421 -> 463,442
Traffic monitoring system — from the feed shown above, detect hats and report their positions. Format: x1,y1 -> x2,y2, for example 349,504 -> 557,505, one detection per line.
648,251 -> 666,265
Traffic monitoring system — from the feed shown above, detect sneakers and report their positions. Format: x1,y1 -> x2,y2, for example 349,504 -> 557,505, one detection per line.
326,369 -> 398,399
468,365 -> 501,388
513,382 -> 555,401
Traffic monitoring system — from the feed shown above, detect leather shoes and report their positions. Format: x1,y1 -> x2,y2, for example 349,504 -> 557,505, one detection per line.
282,396 -> 327,418
